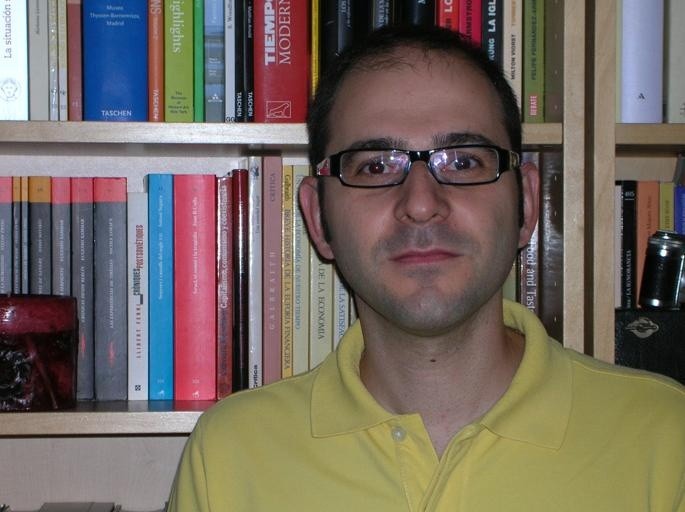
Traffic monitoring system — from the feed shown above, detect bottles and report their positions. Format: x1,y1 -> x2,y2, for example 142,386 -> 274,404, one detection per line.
636,230 -> 685,312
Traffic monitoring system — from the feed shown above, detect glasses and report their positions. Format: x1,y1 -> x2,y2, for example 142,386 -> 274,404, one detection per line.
316,144 -> 521,189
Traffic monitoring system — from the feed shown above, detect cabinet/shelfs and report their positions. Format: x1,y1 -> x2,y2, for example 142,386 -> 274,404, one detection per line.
1,0 -> 685,512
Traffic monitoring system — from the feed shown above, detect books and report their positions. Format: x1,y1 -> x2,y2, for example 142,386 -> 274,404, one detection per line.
1,1 -> 562,123
615,1 -> 685,384
503,148 -> 562,346
1,148 -> 358,401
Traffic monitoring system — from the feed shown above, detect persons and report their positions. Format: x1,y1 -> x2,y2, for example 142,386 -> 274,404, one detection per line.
165,26 -> 685,512
